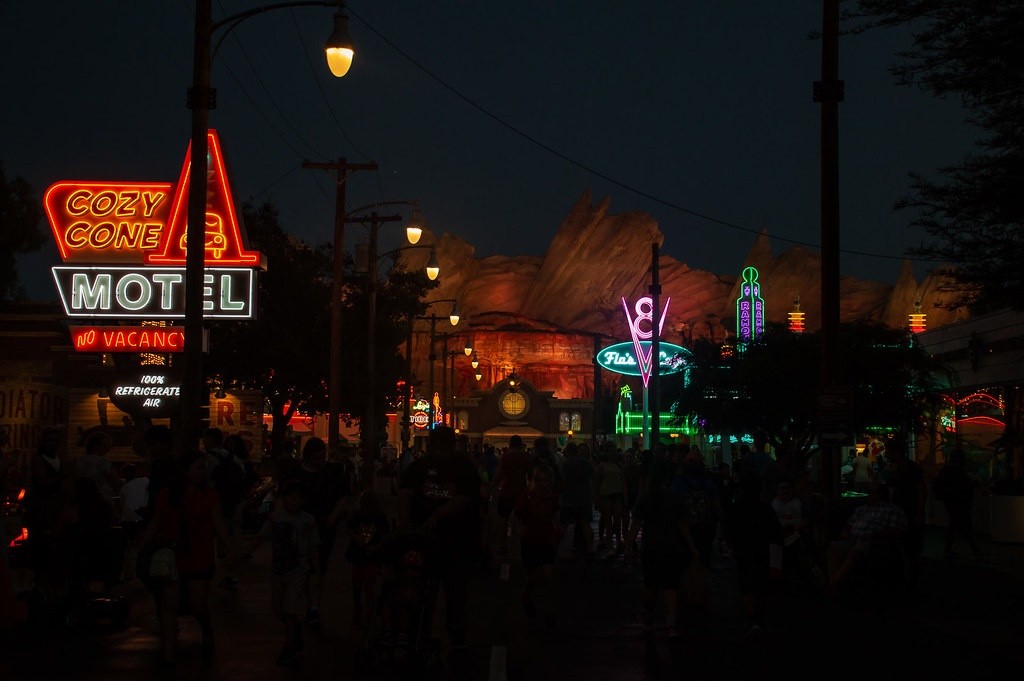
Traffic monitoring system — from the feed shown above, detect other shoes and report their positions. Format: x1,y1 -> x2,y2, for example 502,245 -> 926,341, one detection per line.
745,619 -> 765,640
638,608 -> 680,642
199,630 -> 216,654
571,540 -> 641,566
277,641 -> 307,664
150,659 -> 177,673
521,598 -> 559,634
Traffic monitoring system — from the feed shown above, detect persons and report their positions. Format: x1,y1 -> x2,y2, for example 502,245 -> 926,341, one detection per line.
0,423 -> 1024,664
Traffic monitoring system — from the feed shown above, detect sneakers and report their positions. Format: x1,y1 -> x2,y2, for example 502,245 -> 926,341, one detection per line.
304,607 -> 322,626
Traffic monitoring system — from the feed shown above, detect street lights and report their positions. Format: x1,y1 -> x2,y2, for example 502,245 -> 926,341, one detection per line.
400,299 -> 463,453
364,243 -> 441,479
326,199 -> 426,460
179,0 -> 360,477
420,329 -> 482,452
